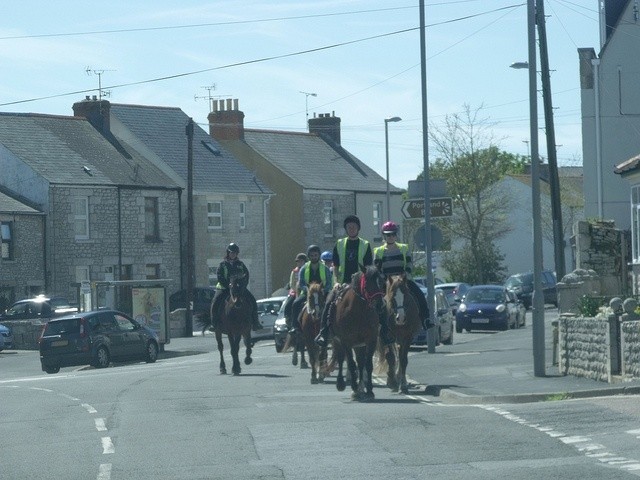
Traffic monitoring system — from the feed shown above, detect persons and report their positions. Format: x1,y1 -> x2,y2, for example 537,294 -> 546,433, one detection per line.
316,215 -> 395,344
288,246 -> 331,334
207,243 -> 264,331
320,250 -> 335,269
373,222 -> 435,330
284,252 -> 309,331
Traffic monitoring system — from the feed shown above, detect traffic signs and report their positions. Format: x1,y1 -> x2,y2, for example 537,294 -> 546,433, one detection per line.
400,197 -> 452,219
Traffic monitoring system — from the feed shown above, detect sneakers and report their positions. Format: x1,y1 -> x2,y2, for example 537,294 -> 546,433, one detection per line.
288,329 -> 294,334
424,320 -> 434,330
253,323 -> 263,331
317,335 -> 326,345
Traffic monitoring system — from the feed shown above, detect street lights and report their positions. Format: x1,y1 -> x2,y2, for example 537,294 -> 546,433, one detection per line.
384,116 -> 402,221
509,59 -> 544,78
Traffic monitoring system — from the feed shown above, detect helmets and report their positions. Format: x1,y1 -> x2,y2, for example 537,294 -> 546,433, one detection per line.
382,221 -> 397,234
308,245 -> 320,258
320,251 -> 332,260
227,243 -> 239,253
344,216 -> 360,230
296,253 -> 307,261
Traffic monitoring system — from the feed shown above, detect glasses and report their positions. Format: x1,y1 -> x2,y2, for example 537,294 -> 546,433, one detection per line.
386,232 -> 396,236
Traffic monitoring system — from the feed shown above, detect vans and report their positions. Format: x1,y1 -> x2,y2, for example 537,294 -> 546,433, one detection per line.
508,272 -> 557,311
39,310 -> 160,373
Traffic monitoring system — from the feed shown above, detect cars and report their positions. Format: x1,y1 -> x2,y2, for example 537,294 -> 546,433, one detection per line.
413,278 -> 444,287
0,324 -> 13,352
244,296 -> 286,348
412,289 -> 454,344
273,295 -> 289,353
456,285 -> 519,333
169,286 -> 216,325
0,297 -> 76,319
433,283 -> 468,315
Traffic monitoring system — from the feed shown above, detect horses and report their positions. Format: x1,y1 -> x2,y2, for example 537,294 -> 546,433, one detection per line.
315,259 -> 387,403
201,270 -> 254,377
375,272 -> 423,394
282,330 -> 298,366
297,279 -> 327,370
